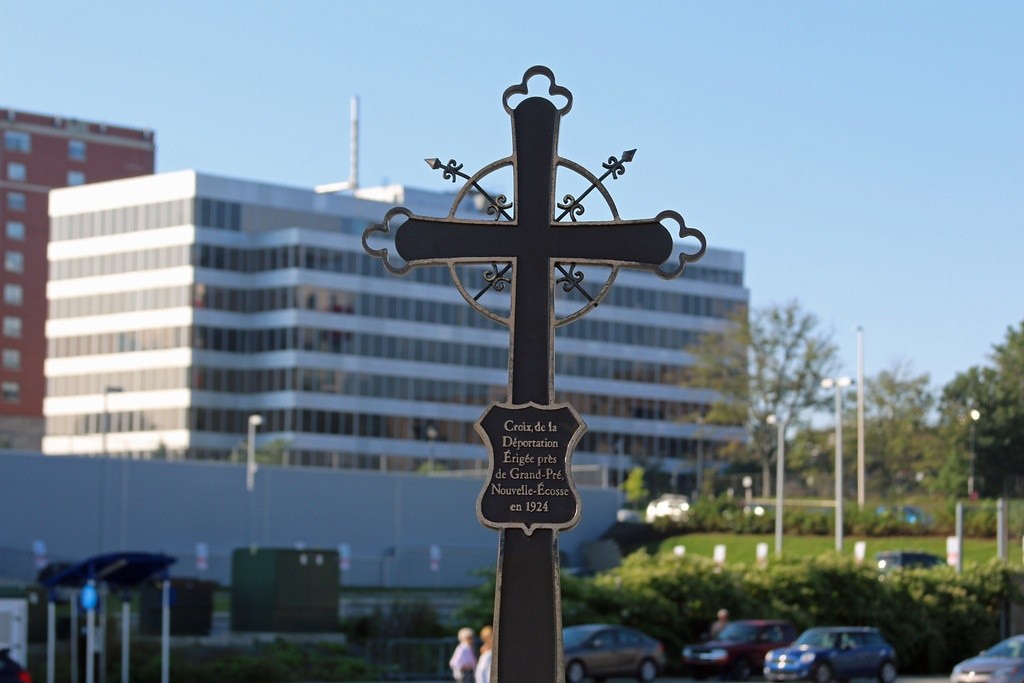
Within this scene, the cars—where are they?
[950,635,1024,683]
[562,624,668,683]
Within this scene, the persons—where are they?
[449,625,493,683]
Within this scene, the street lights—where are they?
[247,414,263,548]
[821,378,852,551]
[767,413,789,563]
[99,385,124,555]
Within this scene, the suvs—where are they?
[682,619,798,682]
[764,625,897,683]
